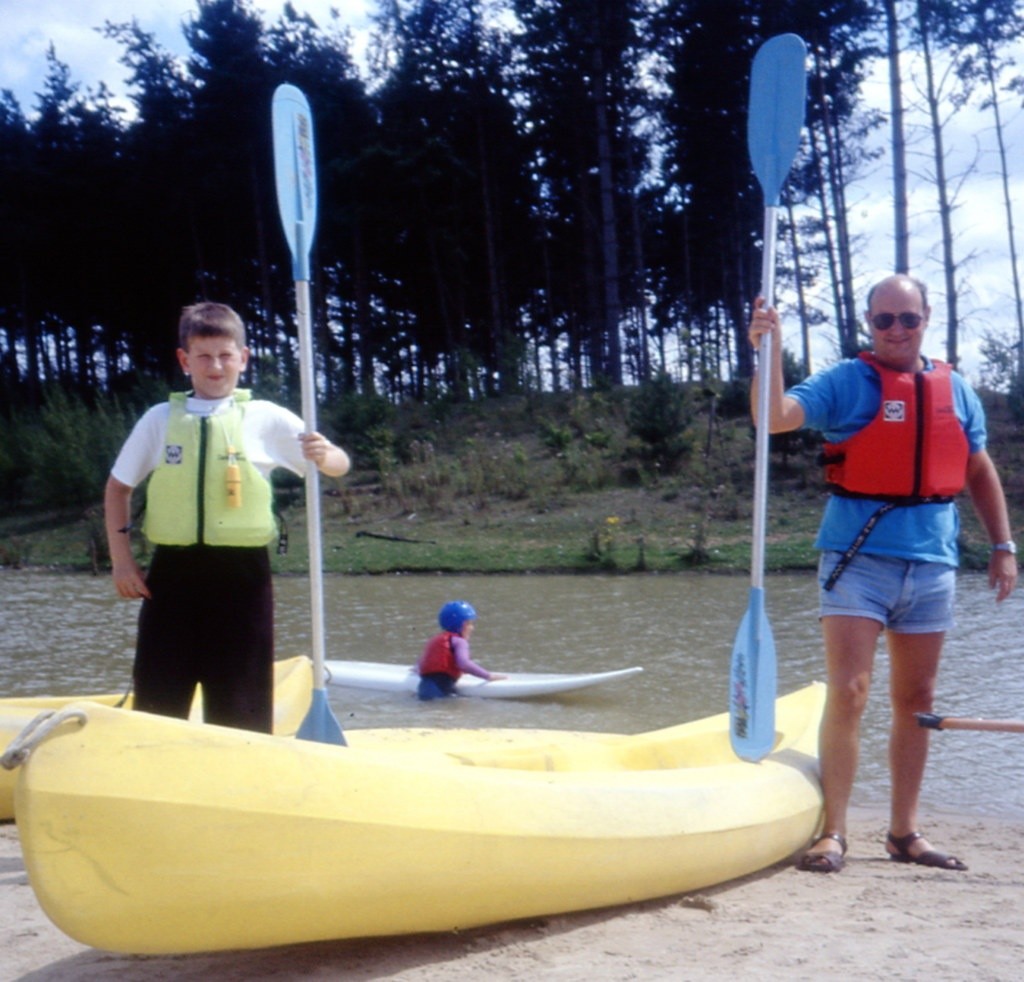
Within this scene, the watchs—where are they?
[991,541,1017,554]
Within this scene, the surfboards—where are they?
[320,653,645,698]
[0,653,316,823]
[0,680,833,957]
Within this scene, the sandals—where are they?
[885,830,969,871]
[796,832,847,872]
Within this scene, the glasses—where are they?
[869,311,925,330]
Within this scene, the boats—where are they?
[322,660,643,698]
[0,679,835,958]
[0,655,316,822]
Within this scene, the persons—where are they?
[105,304,352,736]
[415,599,508,701]
[748,273,1020,876]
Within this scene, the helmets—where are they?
[438,599,475,635]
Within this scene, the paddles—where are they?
[271,82,346,753]
[730,32,809,762]
